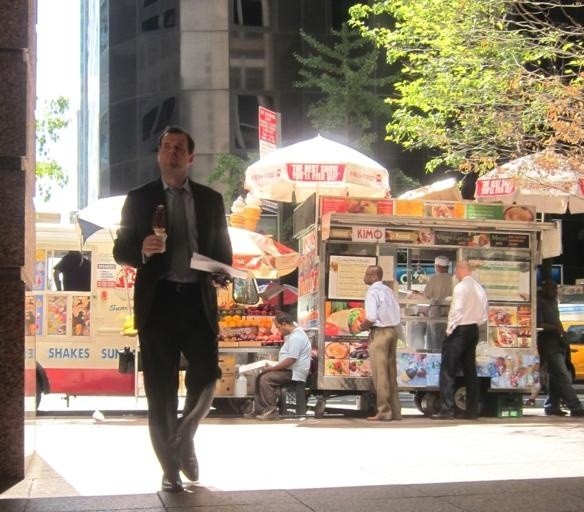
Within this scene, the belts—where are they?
[159,282,200,296]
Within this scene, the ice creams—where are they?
[152,205,167,252]
[230,196,244,229]
[244,192,263,231]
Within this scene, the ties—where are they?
[171,188,190,280]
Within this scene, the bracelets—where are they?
[359,324,364,330]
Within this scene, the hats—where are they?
[435,256,449,266]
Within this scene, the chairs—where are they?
[278,352,314,415]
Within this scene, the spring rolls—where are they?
[328,307,366,335]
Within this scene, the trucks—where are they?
[34,212,273,412]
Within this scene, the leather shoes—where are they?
[162,474,183,492]
[177,418,198,481]
[432,411,455,419]
[455,412,477,419]
[368,415,402,421]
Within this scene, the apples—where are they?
[217,304,276,317]
[218,331,284,346]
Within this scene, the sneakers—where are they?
[243,410,261,419]
[256,409,276,419]
[546,410,567,416]
[571,410,584,416]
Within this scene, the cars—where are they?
[556,303,584,385]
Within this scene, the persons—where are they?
[113,127,232,493]
[430,260,490,420]
[424,256,454,353]
[358,265,402,421]
[537,280,584,416]
[243,312,311,421]
[53,251,90,292]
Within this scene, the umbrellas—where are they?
[474,144,584,242]
[227,226,299,279]
[244,133,391,230]
